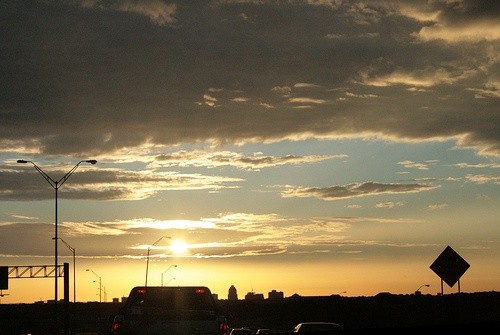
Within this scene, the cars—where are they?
[229,323,344,335]
[111,287,231,335]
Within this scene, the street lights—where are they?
[85,269,107,303]
[162,265,177,287]
[52,237,76,311]
[145,236,171,287]
[16,159,98,335]
